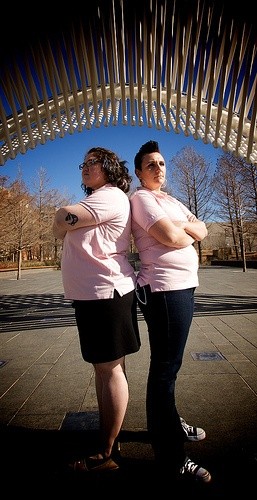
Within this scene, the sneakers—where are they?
[177,456,211,483]
[179,417,206,441]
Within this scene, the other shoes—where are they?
[73,451,112,466]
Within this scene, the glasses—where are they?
[79,159,103,170]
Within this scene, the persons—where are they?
[129,140,212,483]
[52,147,142,473]
